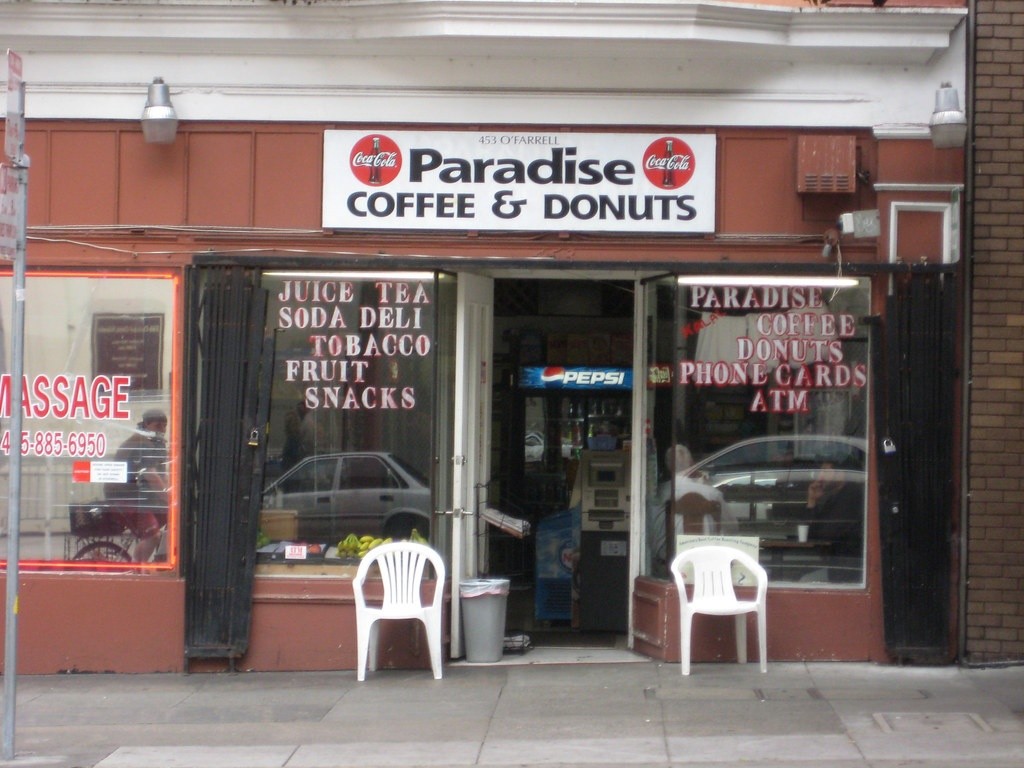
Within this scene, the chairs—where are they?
[671,546,768,675]
[352,542,446,682]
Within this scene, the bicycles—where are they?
[73,486,174,562]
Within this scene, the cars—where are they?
[674,433,868,552]
[262,451,431,538]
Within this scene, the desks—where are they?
[761,537,831,584]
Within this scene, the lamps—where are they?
[140,75,179,144]
[928,81,968,149]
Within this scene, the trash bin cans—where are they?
[459,579,509,664]
